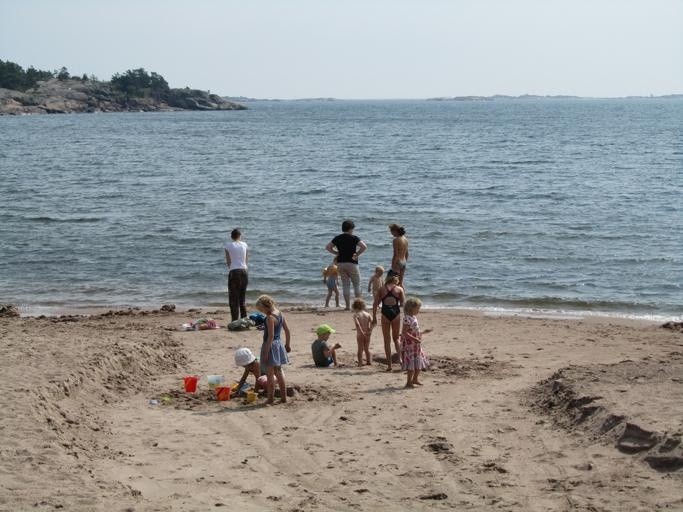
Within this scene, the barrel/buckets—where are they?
[184,376,198,392]
[208,375,224,389]
[247,390,258,402]
[216,385,230,400]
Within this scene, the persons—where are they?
[233,349,278,398]
[255,295,291,404]
[226,229,247,323]
[311,220,430,386]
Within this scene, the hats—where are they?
[316,324,336,336]
[233,347,256,366]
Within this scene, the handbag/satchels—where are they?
[227,312,267,331]
